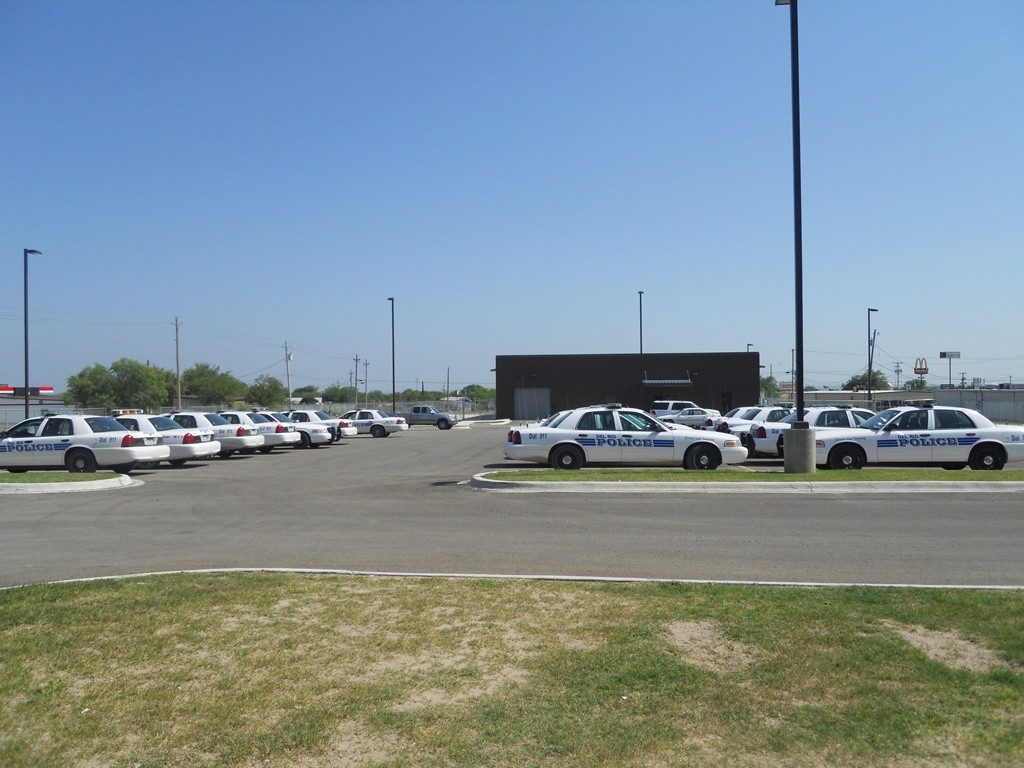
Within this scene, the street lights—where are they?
[867,307,879,400]
[747,344,760,353]
[775,0,813,428]
[22,247,43,420]
[638,291,645,410]
[387,297,395,413]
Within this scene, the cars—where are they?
[110,408,221,469]
[280,410,358,445]
[255,411,336,449]
[215,409,302,453]
[336,408,409,438]
[500,398,1024,472]
[0,411,171,474]
[156,410,265,459]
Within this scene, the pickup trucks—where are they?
[385,403,458,430]
